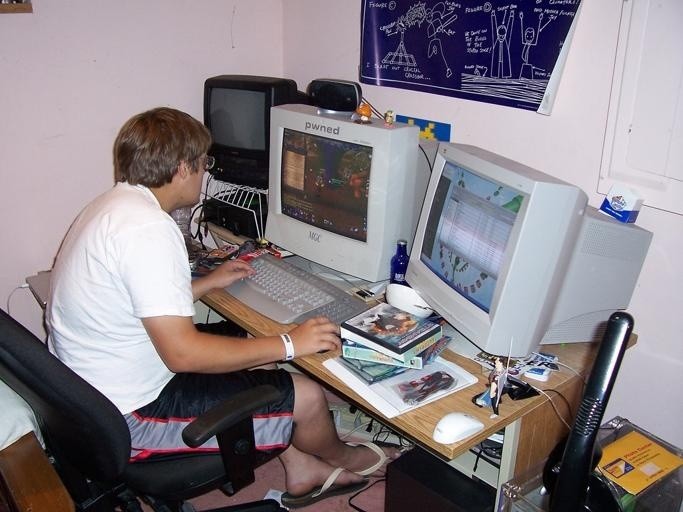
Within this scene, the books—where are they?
[339,356,404,380]
[336,303,445,361]
[339,335,453,369]
[476,350,559,383]
[335,360,407,385]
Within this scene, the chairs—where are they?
[0,306,290,512]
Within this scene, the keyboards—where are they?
[224,253,370,333]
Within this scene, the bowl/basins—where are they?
[384,284,435,319]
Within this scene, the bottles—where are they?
[172,205,191,235]
[390,240,410,288]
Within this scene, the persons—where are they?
[475,355,509,418]
[45,108,400,506]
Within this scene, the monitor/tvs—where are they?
[404,143,653,357]
[204,74,297,192]
[265,104,438,284]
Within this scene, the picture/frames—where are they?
[0,1,33,15]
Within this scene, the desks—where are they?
[190,215,637,512]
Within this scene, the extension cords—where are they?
[331,405,411,446]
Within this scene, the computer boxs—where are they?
[385,447,497,512]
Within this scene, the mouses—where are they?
[432,410,486,446]
[315,331,346,354]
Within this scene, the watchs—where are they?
[278,331,296,361]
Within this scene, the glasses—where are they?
[178,156,216,170]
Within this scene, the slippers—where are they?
[344,440,408,477]
[280,466,370,509]
